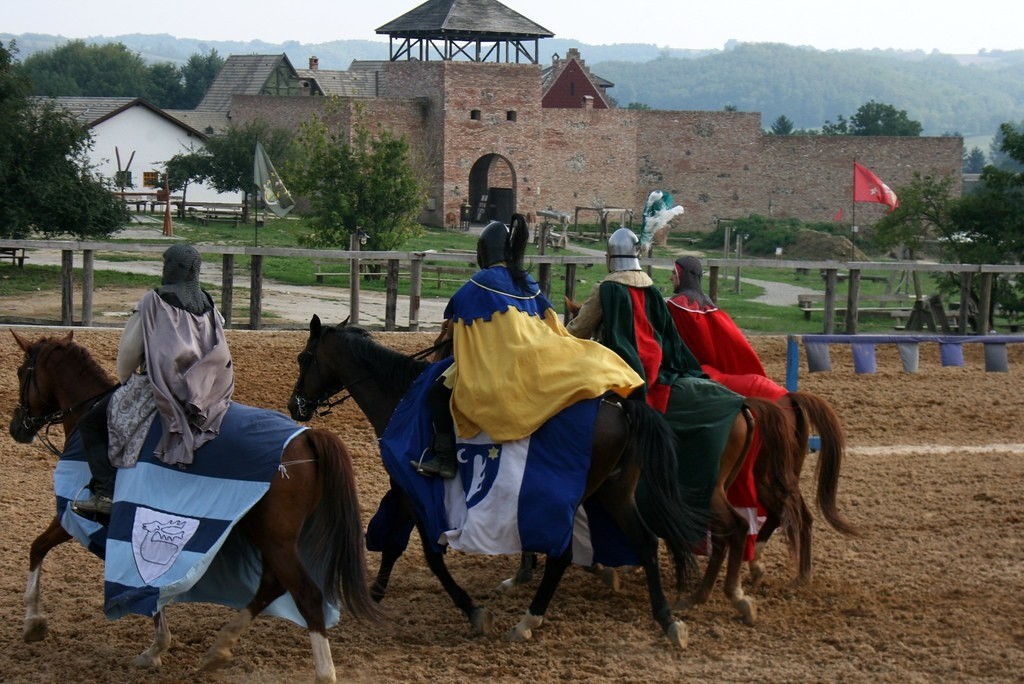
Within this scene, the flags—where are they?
[854,160,899,216]
[254,140,296,220]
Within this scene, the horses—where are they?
[285,298,866,650]
[9,329,390,684]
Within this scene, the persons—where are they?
[565,227,682,399]
[408,221,560,480]
[71,242,234,528]
[664,255,758,395]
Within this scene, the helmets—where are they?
[474,219,513,267]
[606,228,644,276]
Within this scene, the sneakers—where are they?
[67,493,113,518]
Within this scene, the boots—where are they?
[413,430,459,479]
